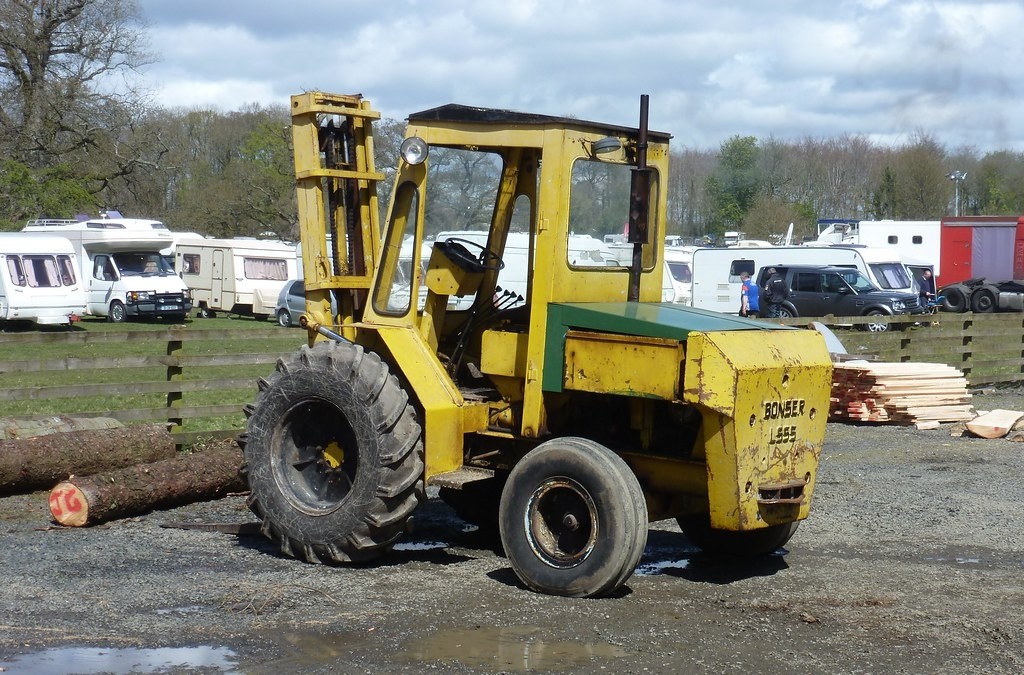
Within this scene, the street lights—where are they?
[945,171,968,217]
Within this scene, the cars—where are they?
[276,280,339,328]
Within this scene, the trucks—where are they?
[22,210,192,324]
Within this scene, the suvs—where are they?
[755,264,922,333]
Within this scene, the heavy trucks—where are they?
[938,216,1024,314]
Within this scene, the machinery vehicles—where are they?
[234,92,833,599]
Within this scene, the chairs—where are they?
[143,262,159,273]
[821,276,827,293]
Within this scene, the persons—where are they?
[763,268,791,318]
[917,270,932,327]
[739,271,759,318]
